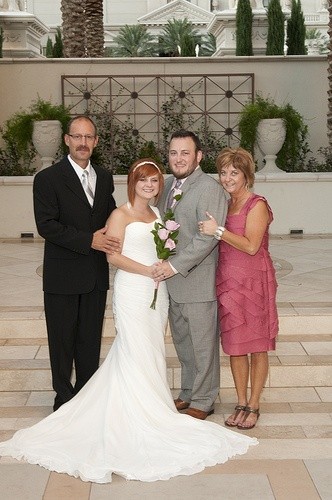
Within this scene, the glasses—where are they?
[67,133,95,141]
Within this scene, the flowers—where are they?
[170,187,184,208]
[149,208,181,310]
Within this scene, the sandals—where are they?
[225,405,247,427]
[238,407,260,430]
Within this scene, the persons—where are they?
[0,158,259,483]
[199,147,279,430]
[33,116,121,411]
[151,130,228,420]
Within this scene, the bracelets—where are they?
[214,226,225,240]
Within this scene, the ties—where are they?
[166,180,181,211]
[82,170,93,198]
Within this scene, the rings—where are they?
[200,228,202,231]
[209,216,212,218]
[200,221,202,224]
[162,273,165,278]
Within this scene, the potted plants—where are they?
[3,92,72,176]
[238,93,308,173]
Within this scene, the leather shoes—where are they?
[187,407,214,420]
[173,398,191,410]
